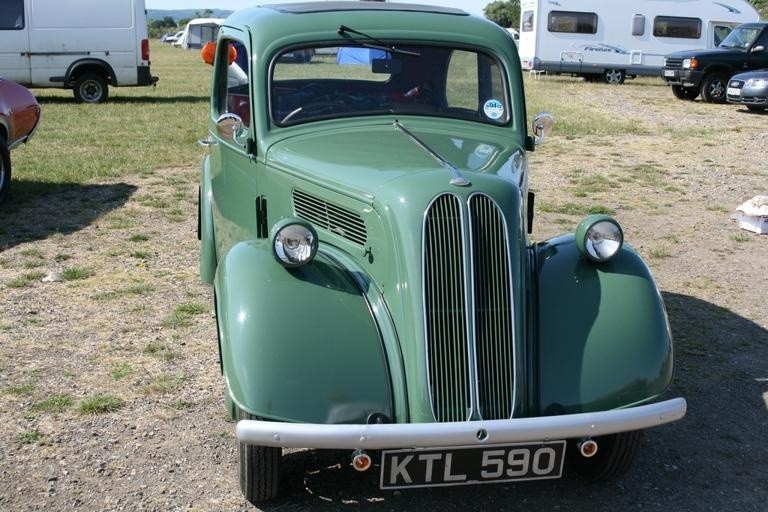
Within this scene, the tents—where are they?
[336,47,394,68]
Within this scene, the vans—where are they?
[517,1,767,87]
[0,0,160,103]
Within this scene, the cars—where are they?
[0,77,41,205]
[660,23,768,112]
[199,1,689,503]
[168,31,185,42]
